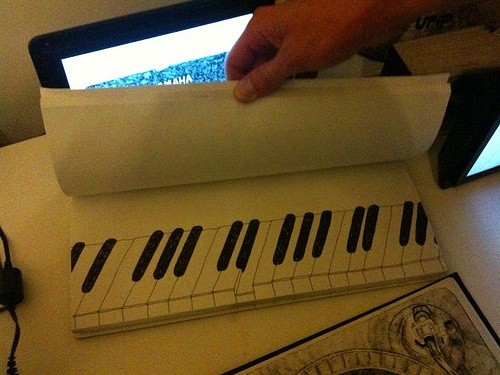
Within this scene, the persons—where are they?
[222,1,470,103]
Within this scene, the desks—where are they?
[2,18,500,375]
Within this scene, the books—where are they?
[34,69,451,343]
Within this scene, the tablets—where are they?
[28,1,279,88]
